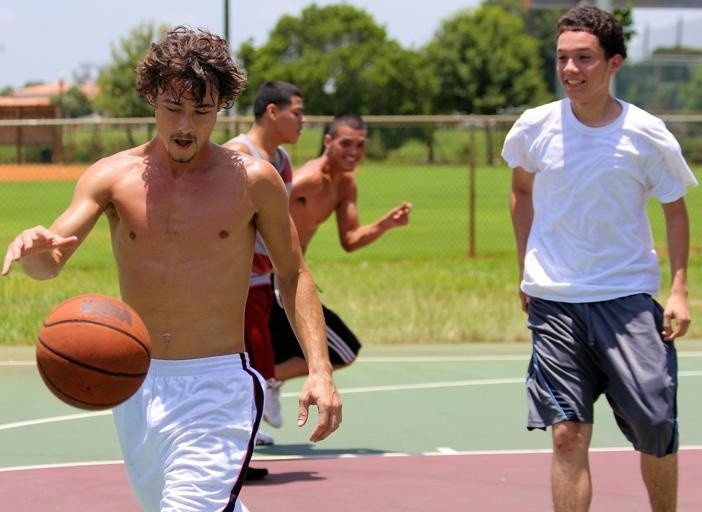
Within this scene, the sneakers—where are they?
[246,378,286,482]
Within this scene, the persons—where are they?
[219,79,306,480]
[254,115,411,445]
[500,5,698,512]
[0,25,342,512]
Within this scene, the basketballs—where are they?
[37,295,151,409]
